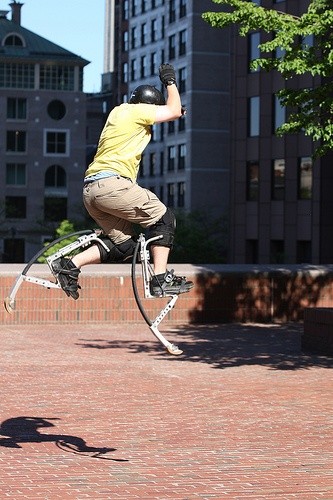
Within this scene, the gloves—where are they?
[159,64,177,88]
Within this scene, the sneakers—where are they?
[51,258,83,300]
[149,268,194,296]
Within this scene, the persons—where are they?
[53,63,193,300]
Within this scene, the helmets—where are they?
[130,85,167,106]
